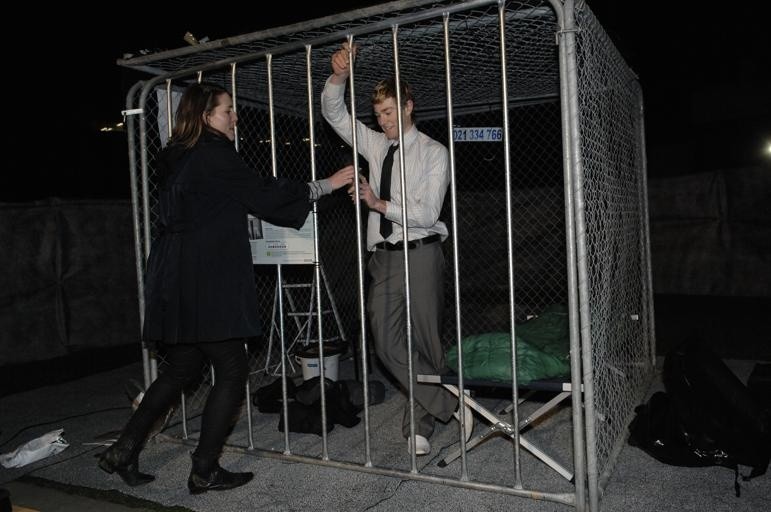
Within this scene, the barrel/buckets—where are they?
[295,342,343,382]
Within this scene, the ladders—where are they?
[263,254,347,376]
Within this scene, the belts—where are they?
[375,236,440,251]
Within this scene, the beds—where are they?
[417,323,642,488]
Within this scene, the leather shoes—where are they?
[408,434,430,456]
[455,388,474,443]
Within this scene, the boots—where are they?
[99,430,156,486]
[189,450,253,495]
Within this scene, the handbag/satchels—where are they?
[629,339,771,497]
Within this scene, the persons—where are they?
[319,43,474,456]
[0,486,40,512]
[97,77,362,496]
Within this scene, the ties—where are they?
[380,143,396,239]
